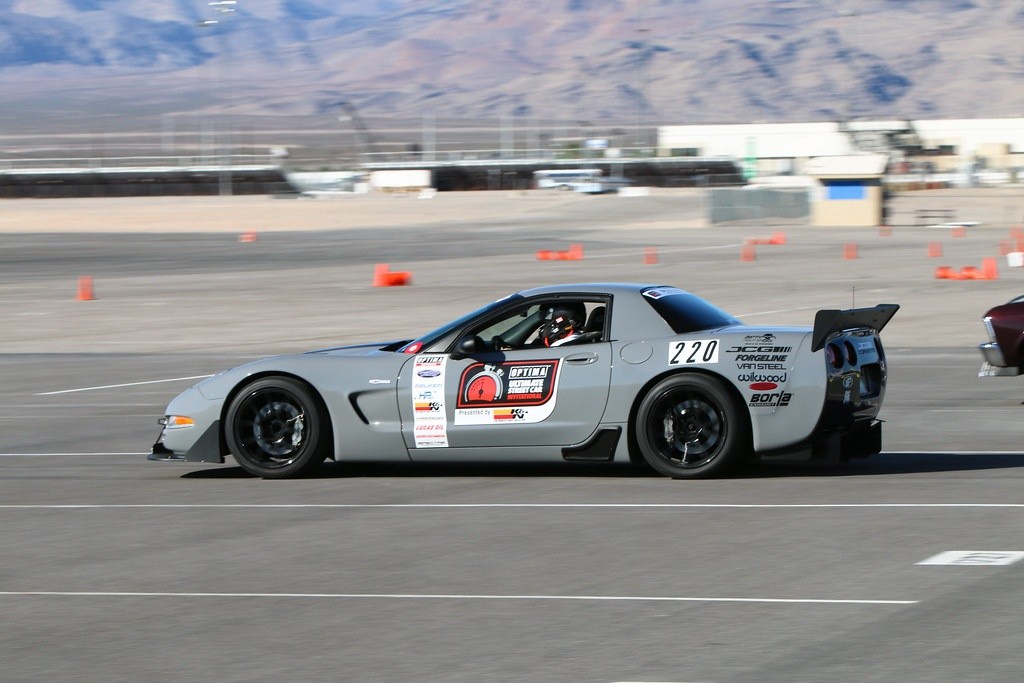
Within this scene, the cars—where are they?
[977,295,1024,378]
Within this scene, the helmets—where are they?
[537,299,586,343]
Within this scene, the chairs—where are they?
[586,306,606,341]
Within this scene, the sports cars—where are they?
[147,283,901,482]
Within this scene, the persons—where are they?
[500,300,586,350]
